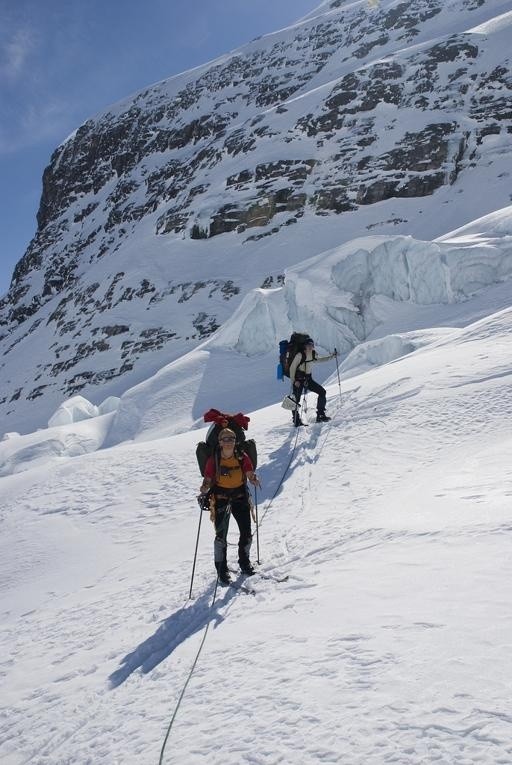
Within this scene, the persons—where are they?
[196,427,262,587]
[289,338,337,426]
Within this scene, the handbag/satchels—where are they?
[281,395,302,411]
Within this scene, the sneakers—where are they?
[317,412,331,422]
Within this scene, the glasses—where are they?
[218,437,235,442]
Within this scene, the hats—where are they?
[218,428,236,446]
[304,339,313,345]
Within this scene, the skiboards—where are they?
[225,569,289,595]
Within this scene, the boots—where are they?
[215,560,234,588]
[293,419,308,427]
[237,559,256,576]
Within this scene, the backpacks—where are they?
[283,332,317,378]
[196,414,257,482]
